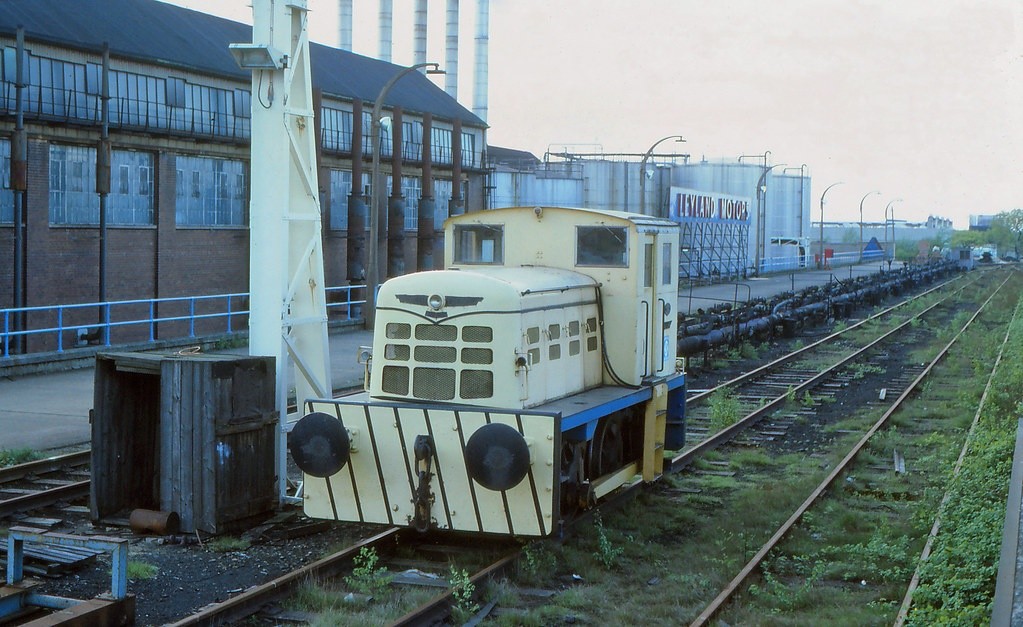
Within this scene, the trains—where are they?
[289,205,684,540]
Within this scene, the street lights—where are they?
[859,190,882,264]
[756,163,789,277]
[819,180,847,268]
[884,198,903,255]
[364,62,447,331]
[638,135,687,215]
